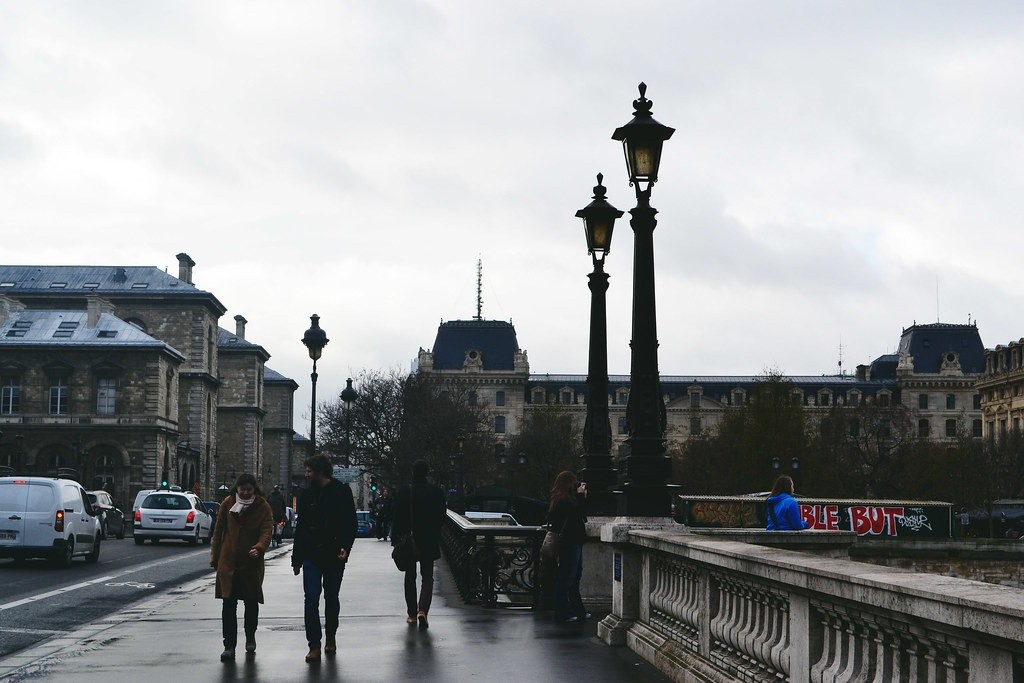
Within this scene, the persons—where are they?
[209,473,274,661]
[389,460,447,628]
[291,453,360,661]
[548,471,592,622]
[766,475,808,530]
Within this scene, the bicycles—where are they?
[272,515,287,547]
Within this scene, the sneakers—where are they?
[324,632,336,653]
[306,648,321,662]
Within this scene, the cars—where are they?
[85,490,126,539]
[356,521,372,538]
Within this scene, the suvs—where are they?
[132,486,221,546]
[0,466,102,569]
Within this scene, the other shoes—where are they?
[583,613,592,619]
[565,616,578,622]
[377,536,381,539]
[245,630,256,651]
[418,609,428,629]
[407,616,417,623]
[384,537,387,540]
[220,646,235,661]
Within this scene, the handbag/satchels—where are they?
[540,531,562,559]
[392,542,414,571]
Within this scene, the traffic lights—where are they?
[371,477,377,491]
[161,471,169,490]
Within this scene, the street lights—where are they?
[457,432,466,515]
[301,313,330,457]
[499,449,526,513]
[576,173,625,516]
[339,378,359,468]
[611,82,676,518]
